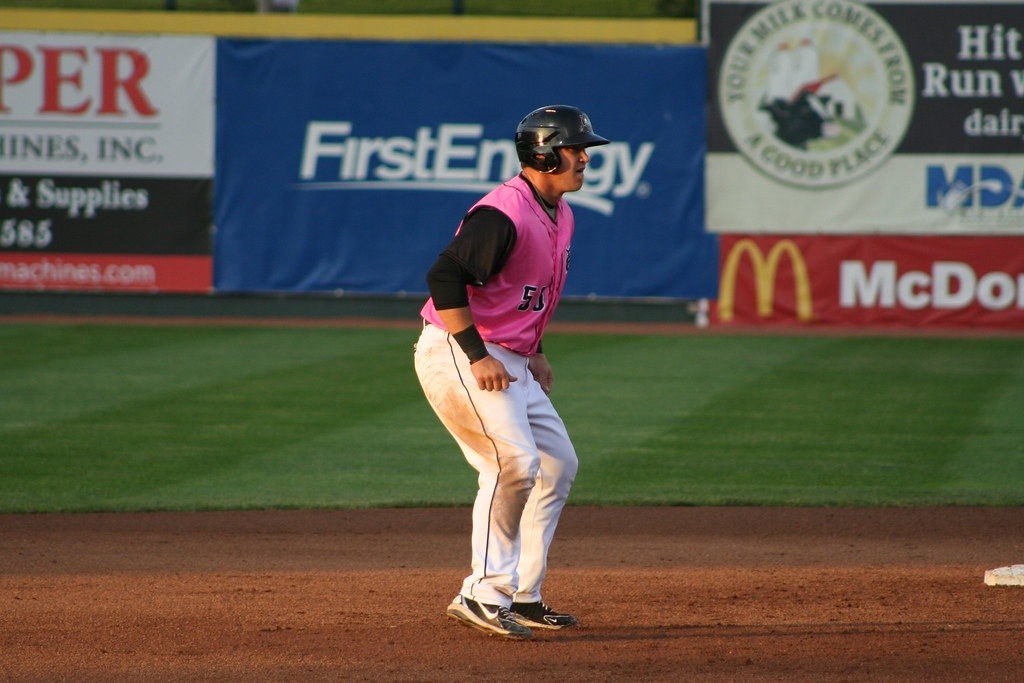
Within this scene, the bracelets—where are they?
[452,323,490,365]
[536,340,543,354]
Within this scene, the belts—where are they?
[424,318,431,326]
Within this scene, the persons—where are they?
[412,104,609,640]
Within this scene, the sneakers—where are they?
[509,600,576,629]
[447,594,532,641]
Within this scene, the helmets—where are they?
[514,104,611,174]
